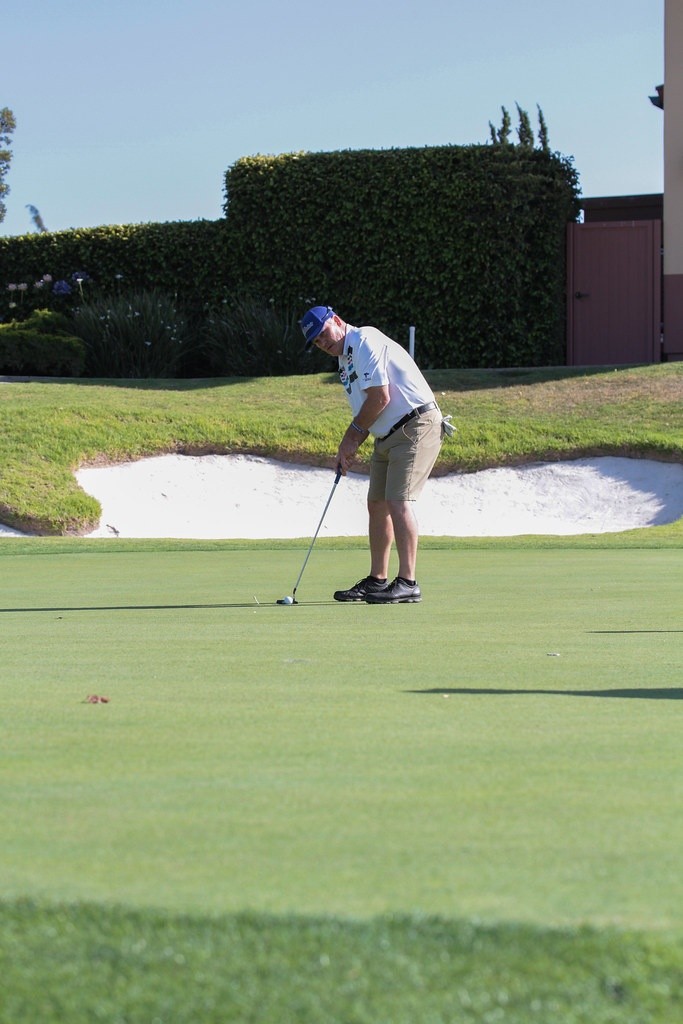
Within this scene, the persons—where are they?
[298,306,455,604]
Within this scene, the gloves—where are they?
[443,414,458,437]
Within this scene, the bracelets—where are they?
[351,422,366,434]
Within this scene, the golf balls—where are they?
[282,596,295,606]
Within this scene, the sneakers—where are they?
[333,577,390,602]
[365,577,424,604]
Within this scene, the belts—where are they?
[381,402,436,441]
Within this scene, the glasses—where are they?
[321,306,333,321]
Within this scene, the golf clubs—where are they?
[277,429,371,606]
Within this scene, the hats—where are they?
[301,305,336,352]
